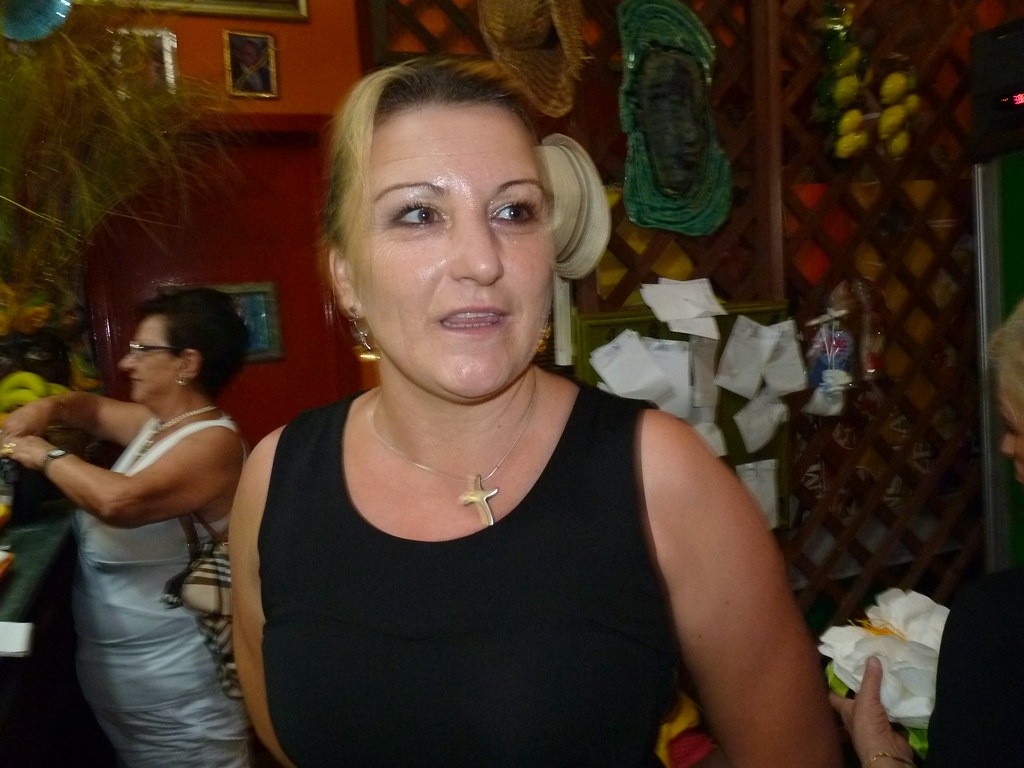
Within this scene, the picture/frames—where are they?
[111,28,177,105]
[71,0,310,25]
[205,282,284,365]
[222,27,280,101]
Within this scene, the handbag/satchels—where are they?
[164,424,257,701]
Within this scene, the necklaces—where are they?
[370,368,540,527]
[132,405,218,462]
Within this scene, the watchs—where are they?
[38,445,73,474]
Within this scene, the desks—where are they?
[0,452,118,662]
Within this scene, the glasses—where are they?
[128,340,184,361]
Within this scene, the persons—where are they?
[825,294,1023,767]
[228,53,854,767]
[0,284,257,767]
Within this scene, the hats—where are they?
[616,0,735,235]
[535,132,611,279]
[477,0,589,117]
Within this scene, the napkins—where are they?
[0,621,34,658]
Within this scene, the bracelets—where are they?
[863,752,914,767]
